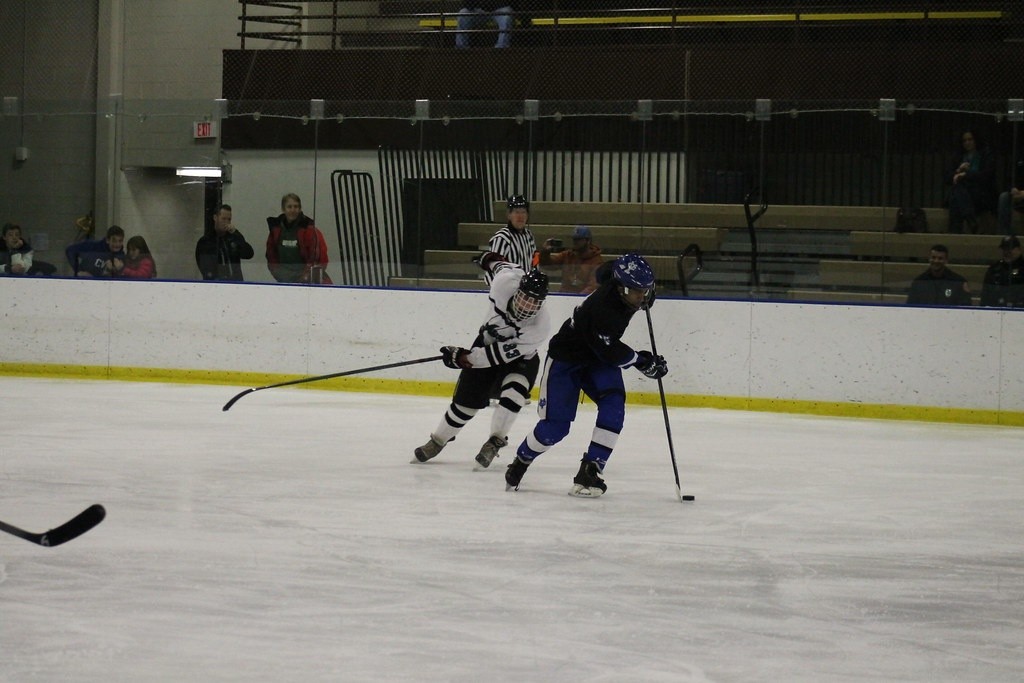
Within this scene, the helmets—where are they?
[613,252,654,309]
[513,270,549,321]
[507,195,529,209]
[573,226,593,240]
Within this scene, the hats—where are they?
[998,235,1020,248]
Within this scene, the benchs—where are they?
[419,10,1001,26]
[387,200,1024,301]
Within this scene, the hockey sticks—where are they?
[222,355,444,412]
[645,304,689,504]
[0,504,107,547]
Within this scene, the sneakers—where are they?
[408,433,456,465]
[471,432,510,473]
[504,452,533,493]
[568,451,607,496]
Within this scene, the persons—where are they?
[65,226,157,278]
[947,129,1024,235]
[455,0,532,48]
[906,244,974,306]
[266,193,333,285]
[195,205,254,282]
[484,195,541,288]
[540,226,605,294]
[505,253,668,494]
[414,250,550,467]
[980,235,1024,308]
[0,224,33,275]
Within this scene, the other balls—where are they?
[682,495,695,501]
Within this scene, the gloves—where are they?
[477,250,504,270]
[439,346,470,370]
[640,350,668,380]
[644,286,655,310]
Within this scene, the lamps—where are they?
[175,165,232,186]
